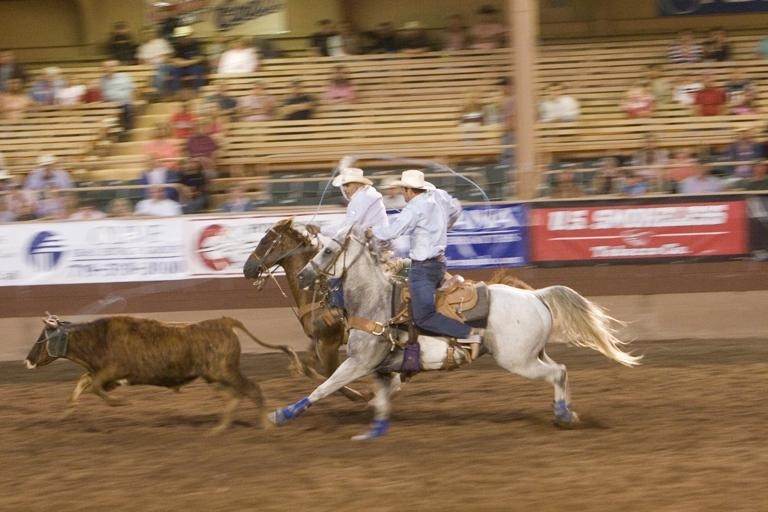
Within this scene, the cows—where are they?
[22,309,304,437]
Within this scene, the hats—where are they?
[36,154,58,168]
[390,170,437,192]
[397,20,427,33]
[491,74,512,88]
[170,25,194,39]
[0,169,16,181]
[331,167,374,187]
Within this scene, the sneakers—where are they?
[468,326,485,360]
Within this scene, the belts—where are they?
[433,254,447,263]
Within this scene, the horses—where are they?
[262,224,645,441]
[242,217,536,403]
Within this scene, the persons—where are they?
[463,27,768,260]
[0,1,354,223]
[303,165,394,248]
[306,5,510,58]
[364,167,484,363]
